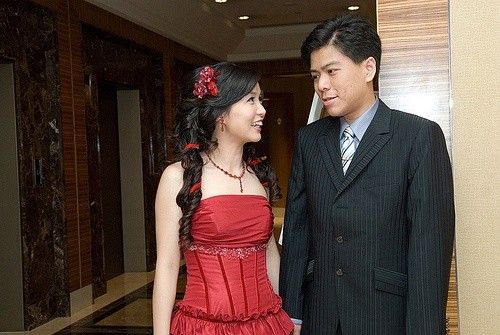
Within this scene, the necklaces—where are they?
[204,150,245,193]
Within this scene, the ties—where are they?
[340,126,356,177]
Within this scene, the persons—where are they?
[279,13,455,335]
[153,61,295,335]
